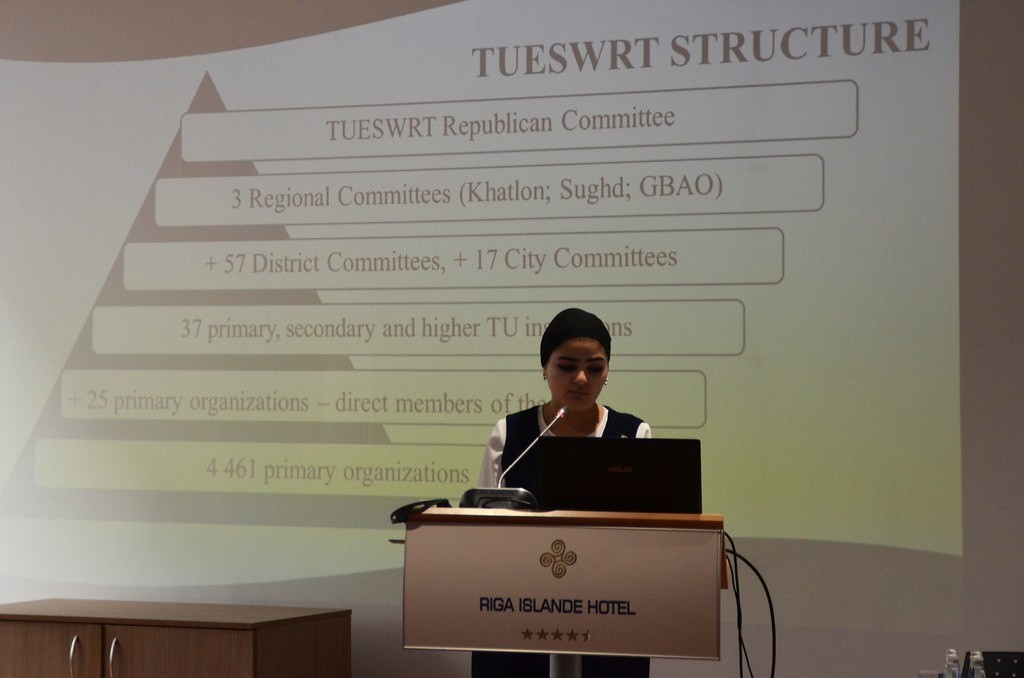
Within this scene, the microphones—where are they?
[459,406,571,512]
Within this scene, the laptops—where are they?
[539,437,702,515]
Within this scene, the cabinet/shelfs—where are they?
[0,598,352,678]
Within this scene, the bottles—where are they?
[967,650,986,678]
[943,648,962,678]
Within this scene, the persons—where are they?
[470,308,651,678]
